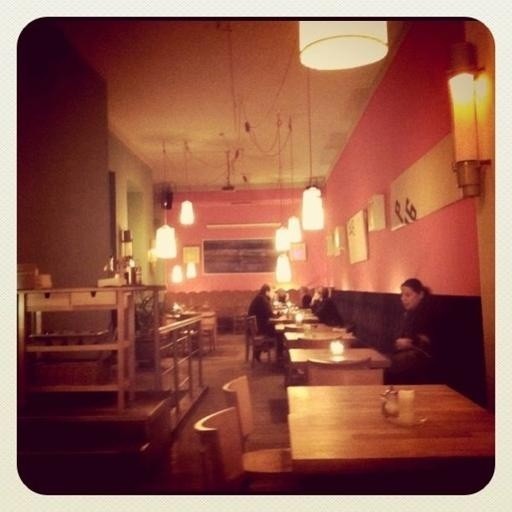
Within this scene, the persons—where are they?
[246,283,284,363]
[274,287,311,309]
[308,286,344,328]
[379,278,445,384]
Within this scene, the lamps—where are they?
[177,140,197,226]
[274,120,293,251]
[153,139,179,260]
[120,229,135,268]
[445,41,487,197]
[299,80,326,232]
[298,20,390,72]
[284,115,303,242]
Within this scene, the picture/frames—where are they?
[200,236,282,274]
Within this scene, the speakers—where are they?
[161,192,173,209]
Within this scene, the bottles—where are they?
[120,256,143,286]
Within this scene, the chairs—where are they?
[194,406,292,495]
[163,307,218,355]
[223,376,289,452]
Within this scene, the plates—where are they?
[387,411,426,427]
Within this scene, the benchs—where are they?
[324,289,489,406]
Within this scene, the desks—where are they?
[17,285,147,410]
[286,384,494,495]
